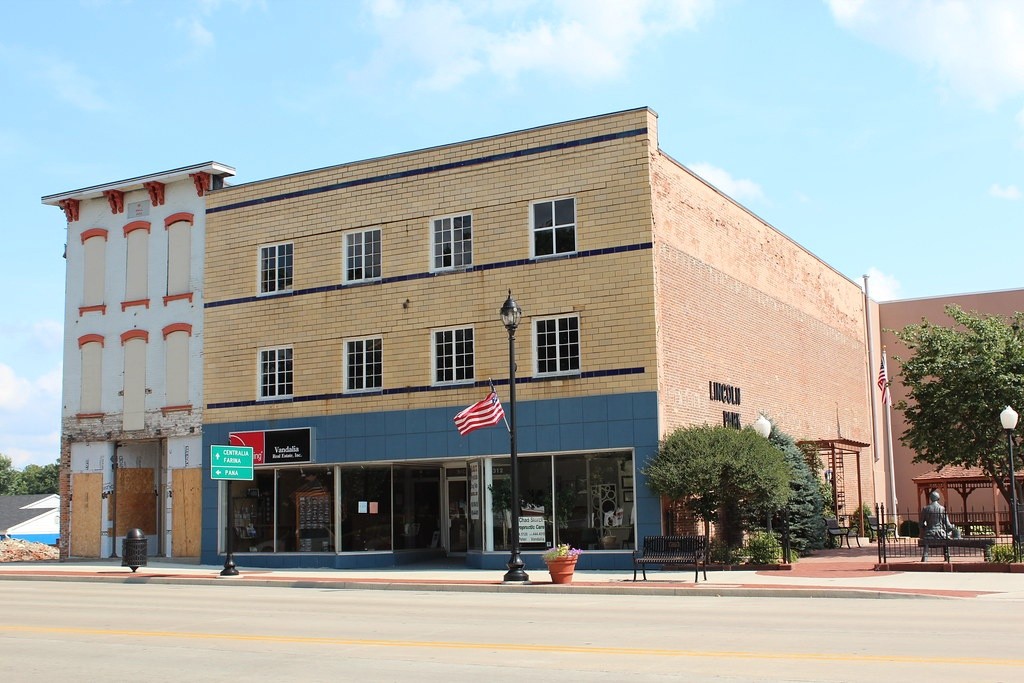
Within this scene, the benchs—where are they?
[633,536,708,583]
[918,538,995,562]
[867,515,898,543]
[823,517,860,549]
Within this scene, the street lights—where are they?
[500,289,529,581]
[1000,405,1020,560]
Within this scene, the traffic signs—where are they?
[210,444,254,480]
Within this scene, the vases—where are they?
[545,555,578,583]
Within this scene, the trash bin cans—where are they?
[121,528,148,573]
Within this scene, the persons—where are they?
[920,492,955,562]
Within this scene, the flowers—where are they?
[542,543,583,561]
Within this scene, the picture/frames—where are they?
[622,476,633,489]
[623,490,634,502]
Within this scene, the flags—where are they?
[877,359,891,407]
[454,391,505,435]
